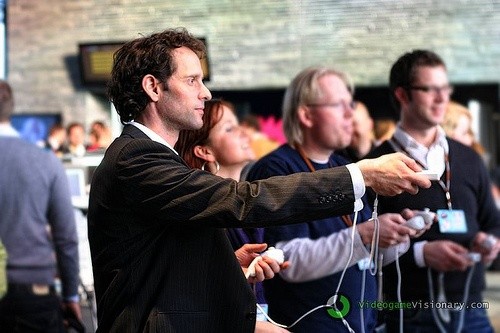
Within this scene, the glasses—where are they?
[410,85,453,94]
[306,99,355,109]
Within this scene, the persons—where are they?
[440,100,489,154]
[44,125,63,152]
[0,75,83,333]
[86,29,431,333]
[247,67,441,333]
[175,81,289,333]
[354,47,500,333]
[66,123,87,156]
[90,122,107,146]
[351,99,382,159]
